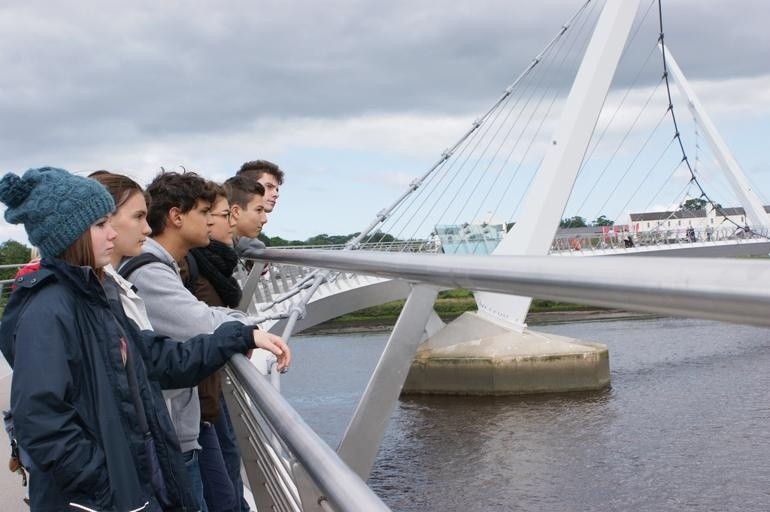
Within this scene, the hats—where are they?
[1,166,116,258]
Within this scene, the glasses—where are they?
[209,212,233,222]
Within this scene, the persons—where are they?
[1,165,292,512]
[175,180,244,512]
[223,177,268,237]
[86,169,172,417]
[118,166,252,512]
[233,160,284,254]
